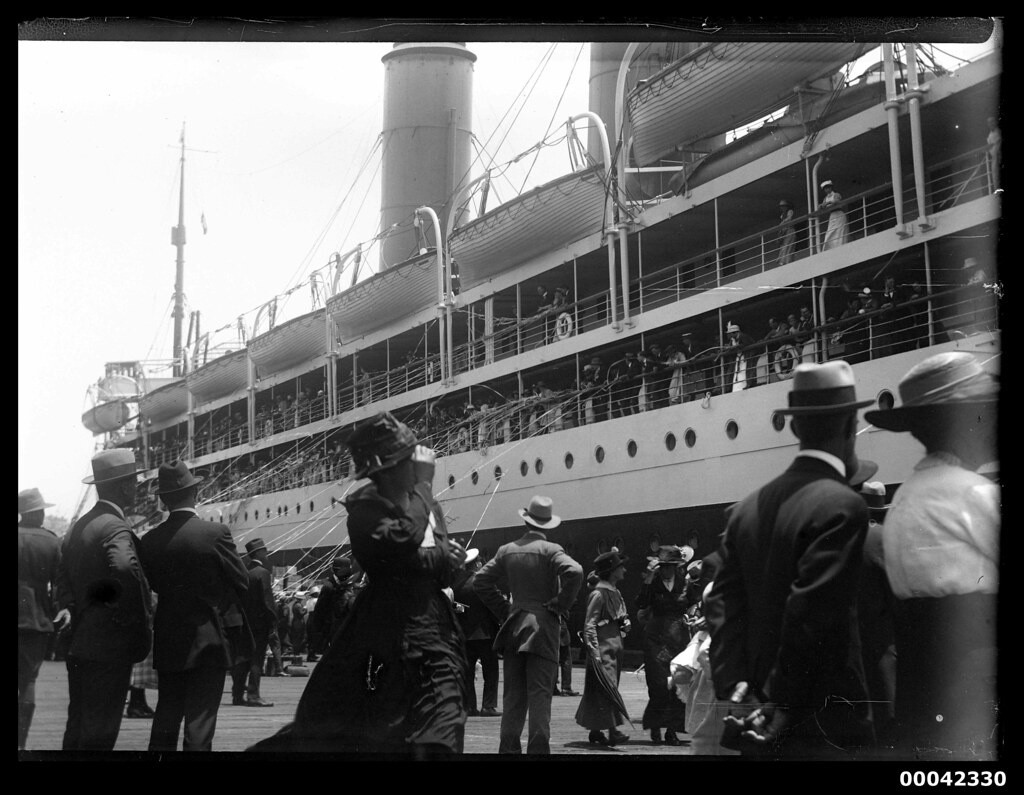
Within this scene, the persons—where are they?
[451,548,508,717]
[863,350,999,759]
[818,180,849,252]
[704,361,875,756]
[845,455,883,562]
[553,611,579,697]
[135,257,994,505]
[986,116,1000,155]
[637,544,740,755]
[230,538,273,707]
[142,459,256,751]
[473,496,584,754]
[577,548,635,748]
[53,446,157,751]
[19,488,60,748]
[777,199,795,266]
[266,558,355,677]
[127,591,157,718]
[244,409,469,755]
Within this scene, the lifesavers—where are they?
[556,312,573,340]
[457,427,469,452]
[774,344,800,381]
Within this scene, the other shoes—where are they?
[276,671,290,676]
[231,694,273,706]
[463,688,681,746]
[127,703,154,718]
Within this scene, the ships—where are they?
[65,41,997,672]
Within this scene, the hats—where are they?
[18,488,56,515]
[718,502,740,535]
[978,462,999,484]
[582,342,677,373]
[685,560,703,582]
[647,544,694,571]
[148,461,204,493]
[308,586,320,595]
[594,551,623,574]
[858,481,893,510]
[843,453,879,485]
[586,570,600,588]
[518,495,561,529]
[726,325,740,334]
[463,548,482,567]
[772,359,875,415]
[81,447,147,483]
[246,537,266,554]
[864,353,1002,432]
[347,412,417,480]
[651,546,686,569]
[328,557,354,572]
[294,592,306,599]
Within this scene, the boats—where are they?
[183,346,258,407]
[136,378,194,426]
[246,306,340,381]
[624,41,885,169]
[445,153,616,294]
[81,396,131,437]
[323,247,443,347]
